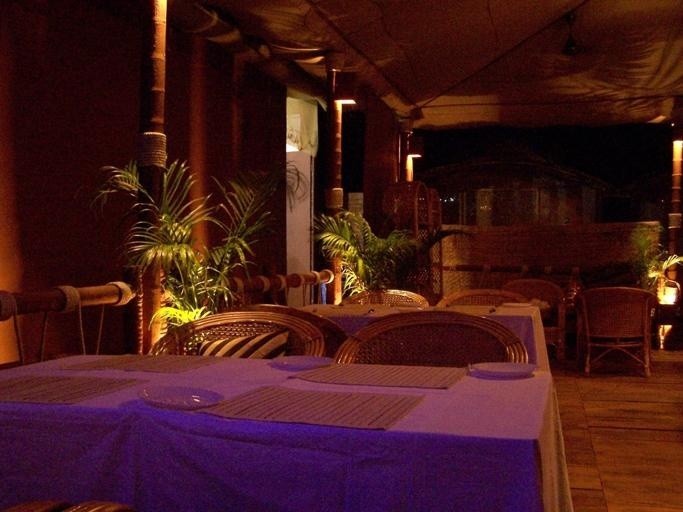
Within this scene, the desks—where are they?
[0,354,577,511]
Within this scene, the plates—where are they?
[468,363,535,378]
[137,385,225,410]
[272,356,332,369]
[503,303,532,307]
